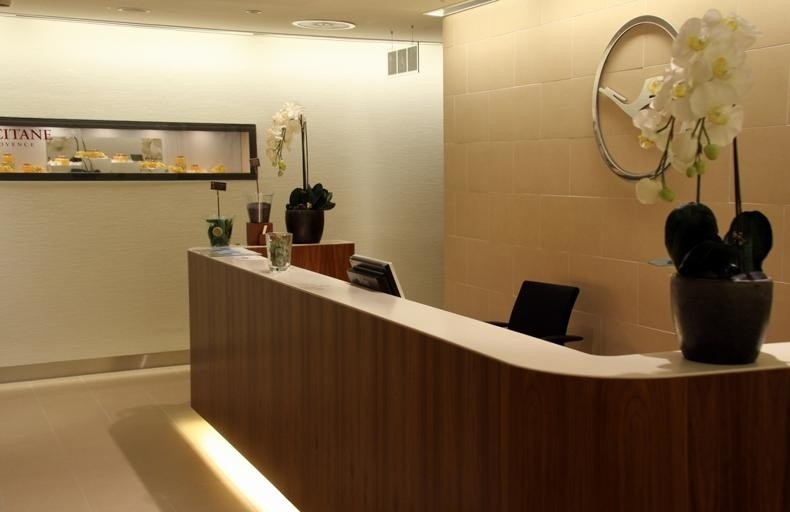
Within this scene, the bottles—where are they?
[175,156,186,173]
[190,164,199,172]
[0,154,15,172]
[22,163,32,172]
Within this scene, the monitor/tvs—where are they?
[345,253,404,297]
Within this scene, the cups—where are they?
[264,231,294,271]
[245,193,272,223]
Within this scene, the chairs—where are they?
[484,280,585,346]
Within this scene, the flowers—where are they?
[264,98,337,209]
[632,7,774,278]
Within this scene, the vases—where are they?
[245,191,276,222]
[668,278,776,366]
[206,218,235,249]
[283,209,325,245]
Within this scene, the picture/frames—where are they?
[0,116,258,181]
